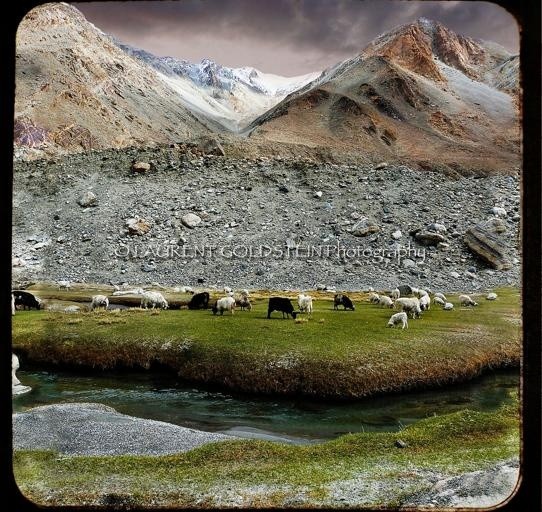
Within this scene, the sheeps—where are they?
[57,279,73,292]
[459,295,479,307]
[140,291,170,310]
[212,286,252,315]
[297,295,313,313]
[387,311,409,332]
[88,295,110,313]
[369,284,448,319]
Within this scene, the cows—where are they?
[11,291,45,311]
[267,297,297,319]
[333,294,355,311]
[187,291,210,310]
